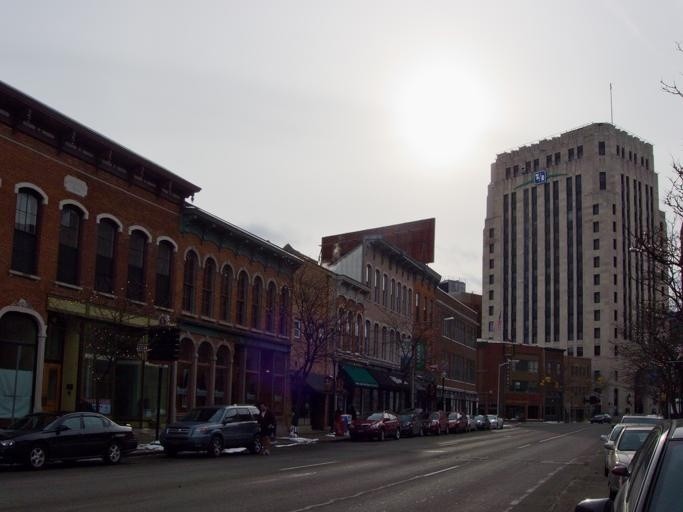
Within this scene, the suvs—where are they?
[158,403,265,456]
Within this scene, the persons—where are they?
[258,402,276,455]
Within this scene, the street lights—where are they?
[497,359,519,417]
[439,370,448,410]
[410,316,454,410]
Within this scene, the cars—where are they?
[0,410,137,473]
[348,406,504,442]
[575,412,683,511]
